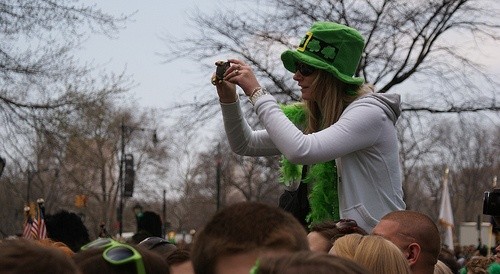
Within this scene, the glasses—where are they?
[336,218,358,235]
[81,237,145,274]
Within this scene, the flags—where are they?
[23,211,34,239]
[32,203,47,239]
[439,180,454,251]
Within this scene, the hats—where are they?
[281,22,365,86]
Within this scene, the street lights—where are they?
[26,168,61,206]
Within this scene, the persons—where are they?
[211,22,407,235]
[0,202,500,274]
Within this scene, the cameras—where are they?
[216,62,231,80]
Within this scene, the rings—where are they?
[237,64,241,70]
[235,69,238,75]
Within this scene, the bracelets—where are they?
[249,87,268,106]
